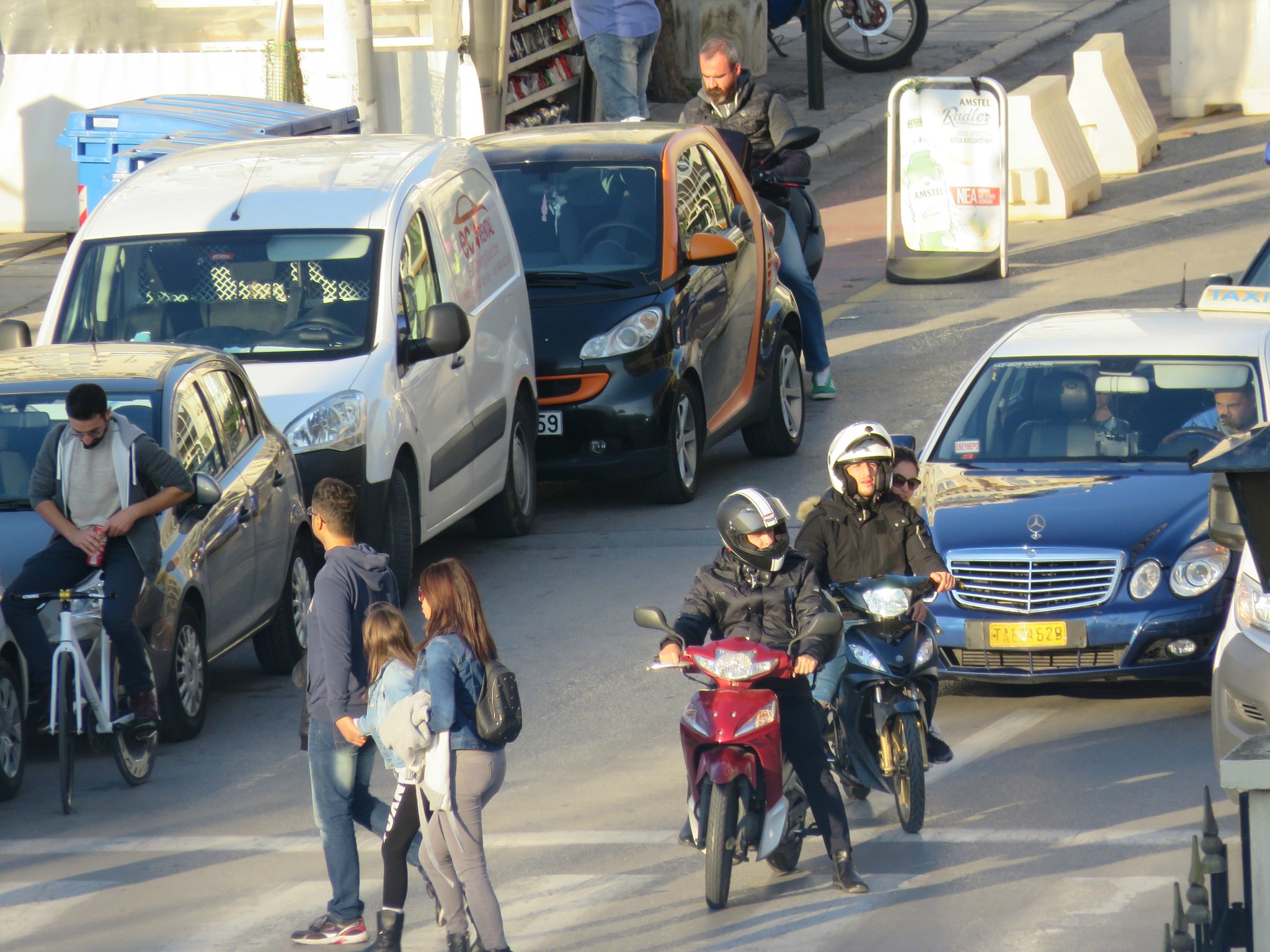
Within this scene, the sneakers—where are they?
[291,914,369,943]
[424,878,448,927]
[811,367,837,400]
[922,730,954,764]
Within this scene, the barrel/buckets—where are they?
[686,793,791,861]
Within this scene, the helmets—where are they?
[716,487,789,571]
[827,421,894,502]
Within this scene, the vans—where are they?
[0,135,540,604]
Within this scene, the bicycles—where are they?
[10,570,160,815]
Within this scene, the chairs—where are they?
[209,247,286,335]
[121,254,187,338]
[1008,372,1113,456]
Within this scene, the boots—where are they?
[361,910,404,951]
[465,906,488,952]
[678,816,695,845]
[447,931,470,952]
[831,851,869,893]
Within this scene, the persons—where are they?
[570,0,662,122]
[657,489,867,892]
[1081,365,1139,458]
[0,384,195,740]
[679,40,837,399]
[1182,382,1258,445]
[793,422,957,765]
[289,477,512,952]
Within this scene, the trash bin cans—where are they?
[54,94,361,228]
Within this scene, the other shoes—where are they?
[130,692,159,740]
[34,696,87,730]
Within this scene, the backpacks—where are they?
[442,628,524,743]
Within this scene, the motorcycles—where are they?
[767,0,930,73]
[633,605,844,909]
[811,569,965,834]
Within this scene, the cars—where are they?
[465,120,808,506]
[1,341,316,745]
[0,611,30,801]
[1203,236,1270,285]
[888,285,1270,697]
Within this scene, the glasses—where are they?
[69,413,106,437]
[418,586,425,601]
[306,507,326,525]
[891,473,922,491]
